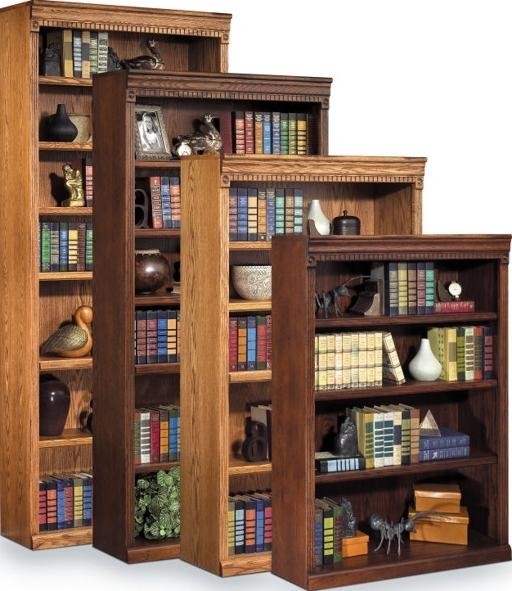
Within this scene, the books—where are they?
[229,489,274,555]
[82,151,95,207]
[38,218,96,275]
[36,471,93,534]
[232,109,314,157]
[314,262,493,473]
[134,174,181,467]
[228,313,274,374]
[314,506,342,568]
[228,184,304,243]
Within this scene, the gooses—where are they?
[120,33,166,70]
[176,112,224,152]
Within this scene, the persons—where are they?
[61,164,85,202]
[62,28,109,80]
[141,113,163,152]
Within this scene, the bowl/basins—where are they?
[231,265,272,301]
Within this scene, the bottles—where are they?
[39,374,72,437]
[406,335,443,383]
[135,246,171,293]
[306,198,331,235]
[48,102,79,142]
